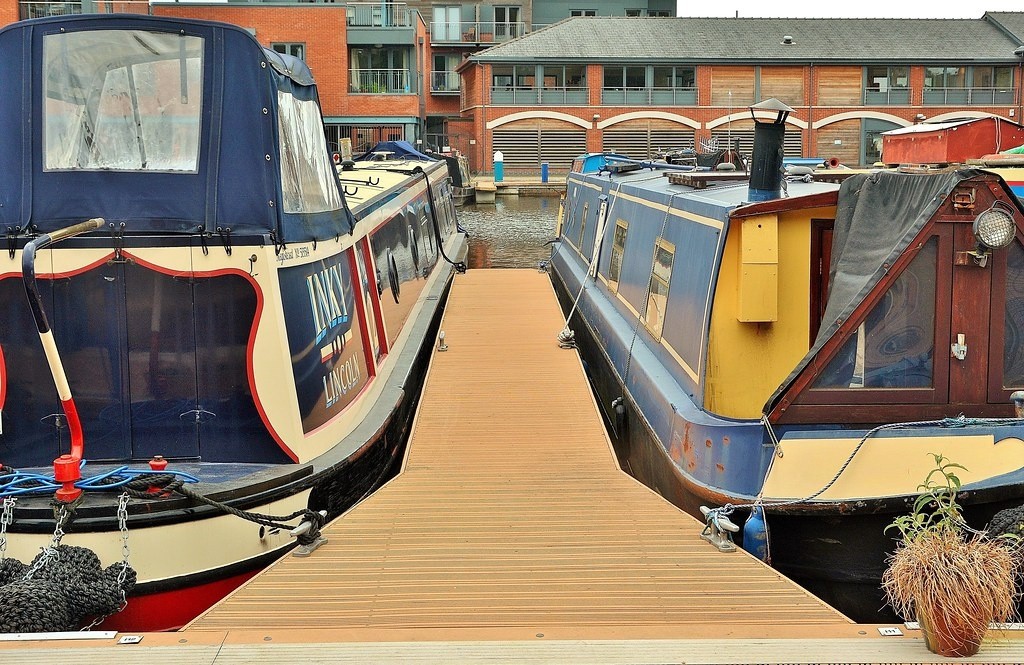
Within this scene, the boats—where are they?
[1,11,469,630]
[547,97,1022,588]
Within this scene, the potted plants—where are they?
[878,453,1024,657]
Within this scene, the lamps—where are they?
[972,200,1016,256]
